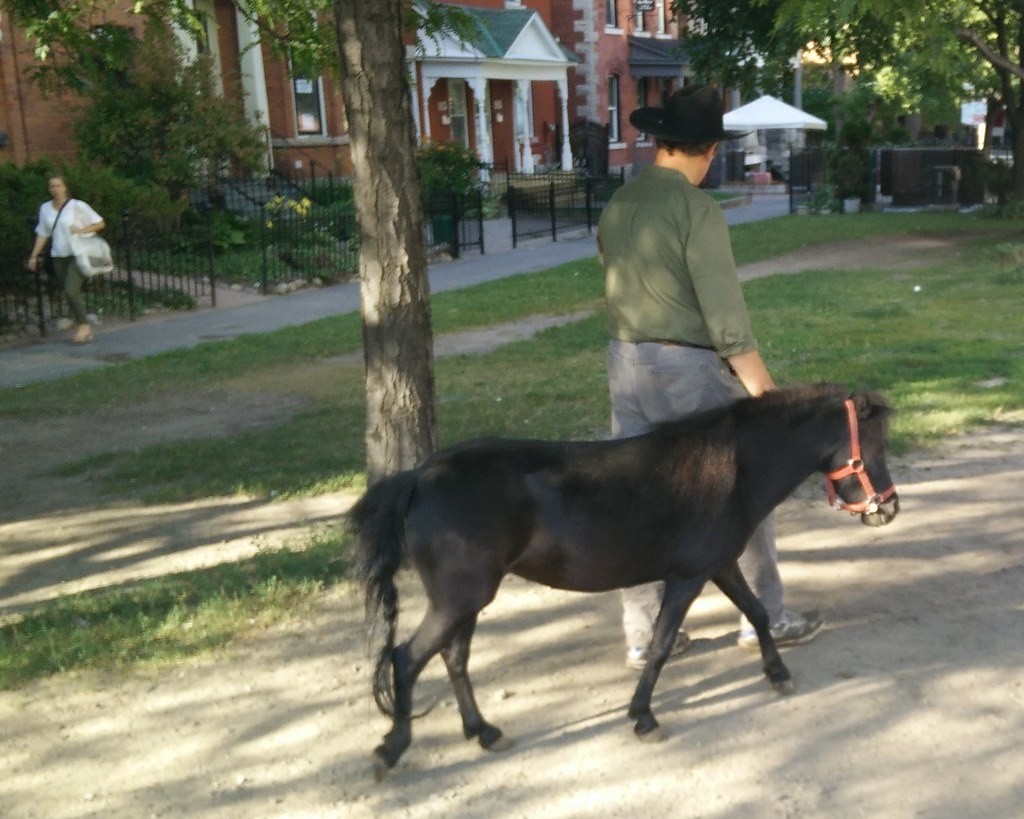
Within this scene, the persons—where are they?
[593,84,908,669]
[25,173,113,344]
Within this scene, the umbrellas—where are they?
[723,94,828,131]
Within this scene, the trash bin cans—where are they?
[931,165,961,204]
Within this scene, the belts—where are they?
[653,339,718,351]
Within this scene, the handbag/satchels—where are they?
[70,199,114,277]
[43,237,53,277]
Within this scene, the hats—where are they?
[629,83,755,144]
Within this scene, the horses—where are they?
[346,379,900,781]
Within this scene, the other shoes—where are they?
[74,328,95,343]
[58,317,74,331]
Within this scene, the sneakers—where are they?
[738,604,827,650]
[626,629,690,669]
